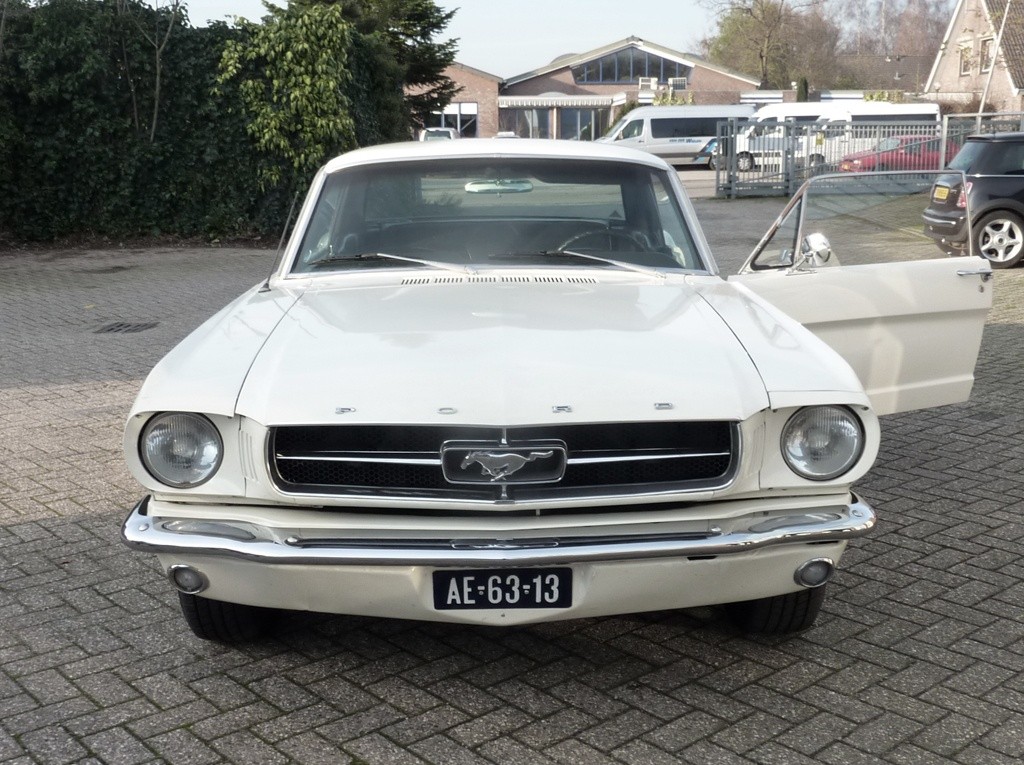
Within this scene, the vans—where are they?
[420,128,460,142]
[592,106,755,169]
[732,102,939,178]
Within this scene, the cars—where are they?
[121,137,994,646]
[492,131,519,138]
[839,135,958,176]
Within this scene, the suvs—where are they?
[921,133,1024,271]
